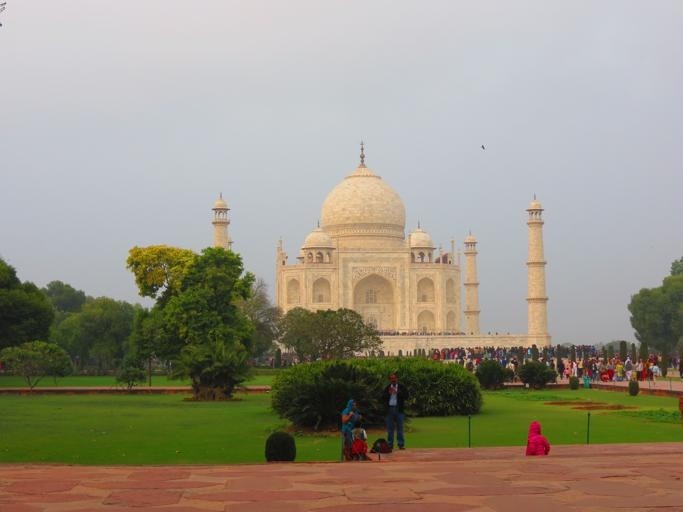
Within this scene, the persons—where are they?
[523,420,550,456]
[430,343,683,383]
[383,373,409,449]
[337,398,361,462]
[349,421,368,460]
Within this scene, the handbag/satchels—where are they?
[372,438,392,453]
